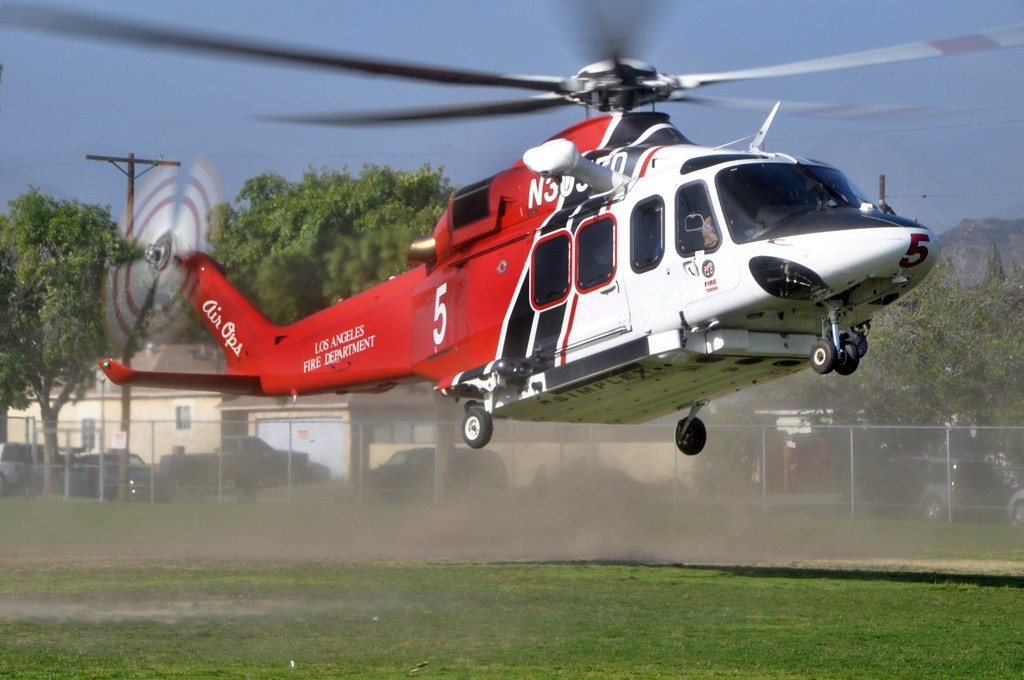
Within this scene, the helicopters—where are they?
[1,0,1024,455]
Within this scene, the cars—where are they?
[362,447,507,497]
[0,443,152,494]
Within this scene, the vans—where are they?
[893,460,1005,519]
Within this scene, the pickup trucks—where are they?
[158,434,310,487]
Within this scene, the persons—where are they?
[654,237,688,261]
[701,217,719,247]
